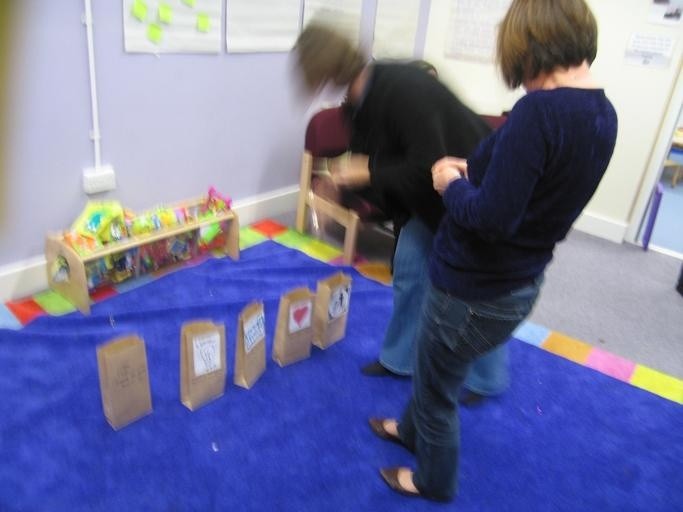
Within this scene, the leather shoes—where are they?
[379,467,422,496]
[362,362,390,376]
[370,415,403,444]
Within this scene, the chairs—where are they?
[295,107,391,268]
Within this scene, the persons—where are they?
[292,21,510,406]
[368,0,617,503]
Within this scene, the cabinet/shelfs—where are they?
[45,197,240,314]
